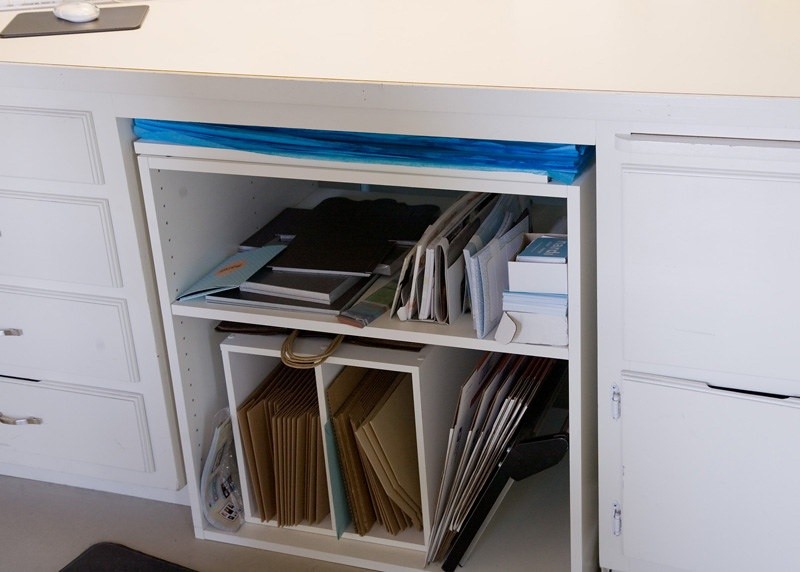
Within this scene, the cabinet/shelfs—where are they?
[0,96,800,572]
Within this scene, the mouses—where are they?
[54,3,100,23]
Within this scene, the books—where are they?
[172,183,570,347]
[200,416,245,533]
